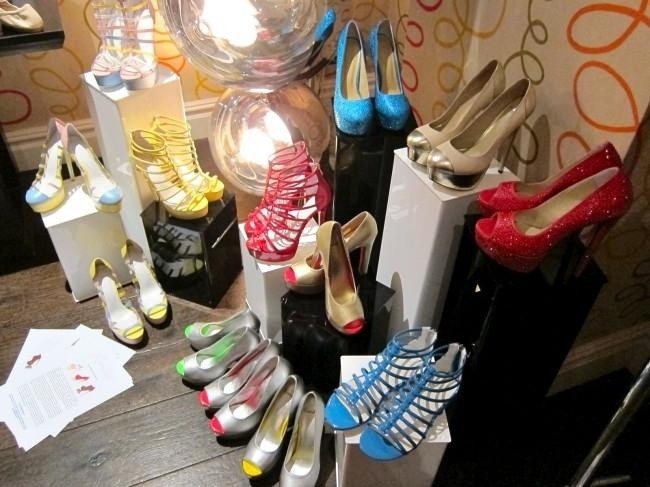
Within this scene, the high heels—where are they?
[25,116,75,212]
[148,114,224,202]
[474,165,634,277]
[427,77,537,191]
[309,220,365,333]
[129,128,209,220]
[245,140,315,239]
[478,140,624,216]
[242,372,325,487]
[283,210,378,293]
[246,162,332,261]
[61,123,124,213]
[407,59,506,166]
[199,338,293,439]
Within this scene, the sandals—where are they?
[90,256,145,345]
[121,238,168,324]
[175,307,261,384]
[325,326,468,461]
[91,0,157,90]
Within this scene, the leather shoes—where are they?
[367,18,410,130]
[333,18,374,134]
